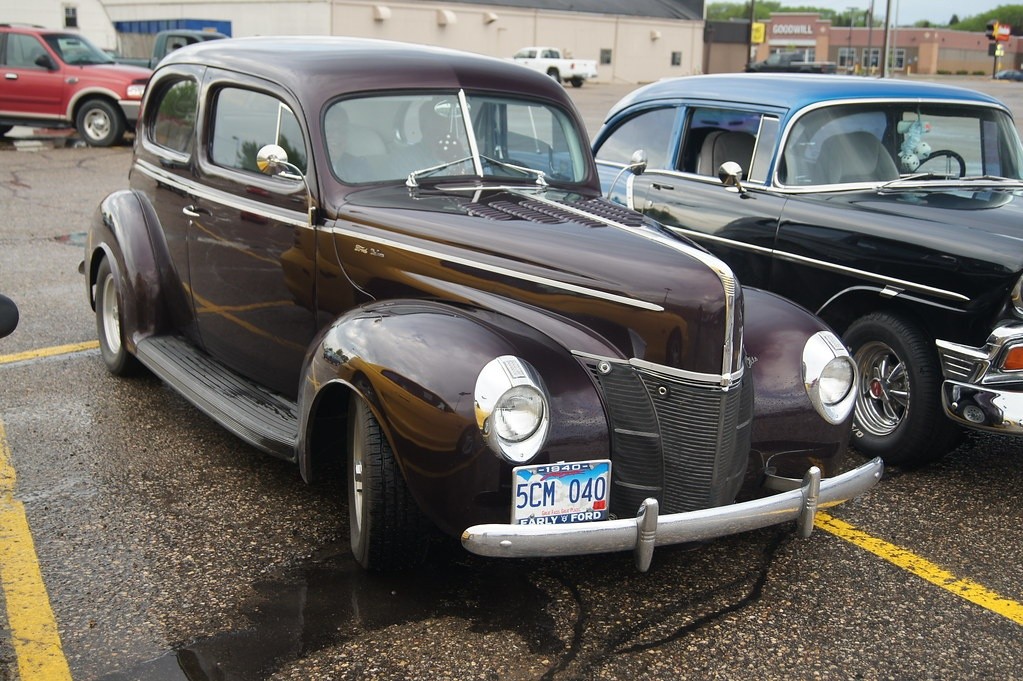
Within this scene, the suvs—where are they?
[0,21,177,148]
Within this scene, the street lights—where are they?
[846,6,859,67]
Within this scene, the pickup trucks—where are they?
[28,26,233,117]
[743,51,838,74]
[502,45,599,87]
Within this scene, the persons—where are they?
[324,104,375,181]
[391,100,494,179]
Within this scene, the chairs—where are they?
[694,130,757,185]
[810,130,900,184]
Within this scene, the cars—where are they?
[451,67,1023,472]
[77,31,887,584]
[989,69,1023,82]
